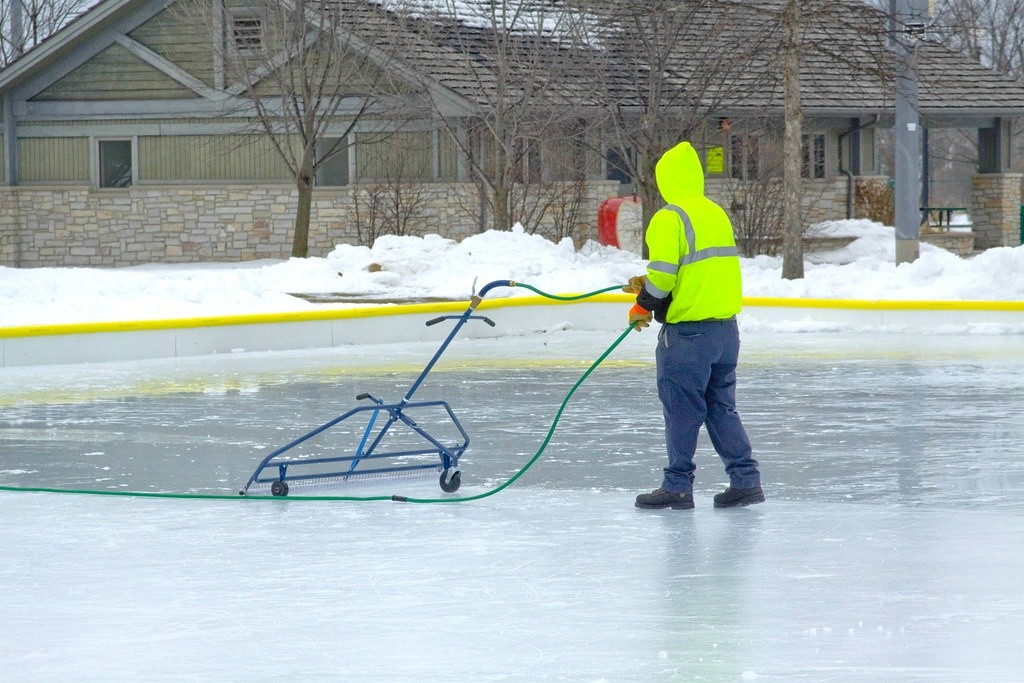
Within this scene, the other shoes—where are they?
[635,487,694,510]
[713,485,765,509]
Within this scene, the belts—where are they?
[701,315,738,321]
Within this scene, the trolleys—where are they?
[235,307,498,497]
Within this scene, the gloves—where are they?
[622,275,647,295]
[629,304,653,333]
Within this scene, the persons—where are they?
[622,141,765,510]
